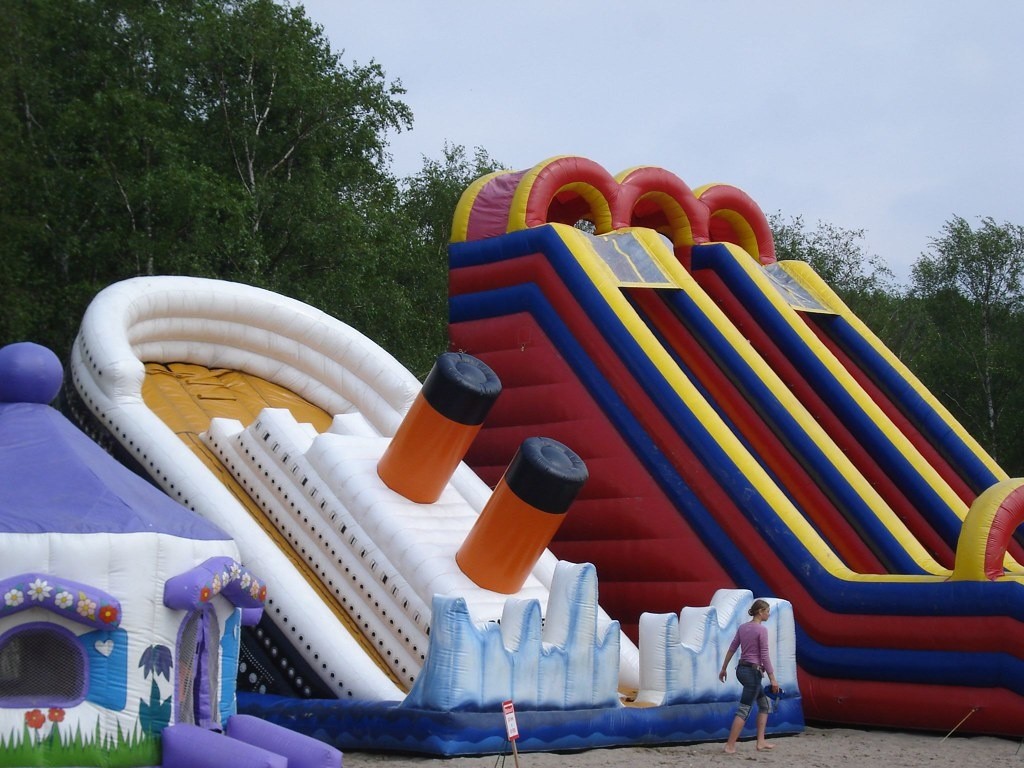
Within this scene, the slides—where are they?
[65,276,807,755]
[441,155,1023,739]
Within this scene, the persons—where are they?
[719,599,780,755]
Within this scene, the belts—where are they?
[738,660,766,680]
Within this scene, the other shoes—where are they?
[764,685,785,702]
